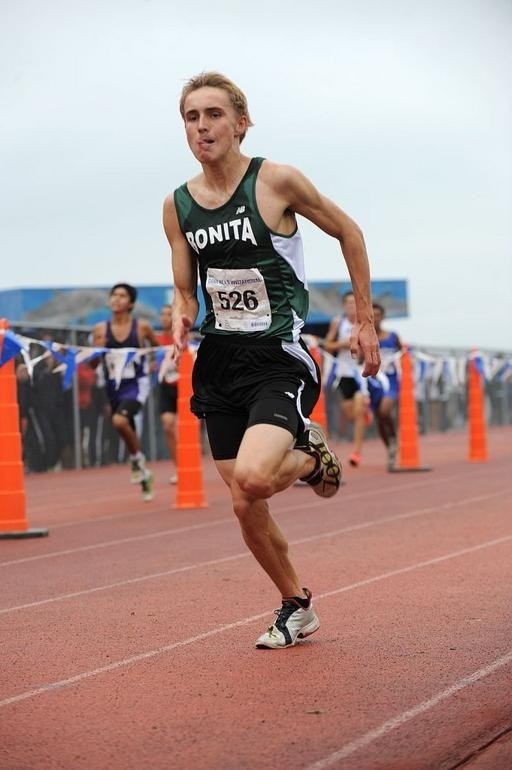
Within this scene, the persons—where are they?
[164,72,381,649]
[0,284,512,501]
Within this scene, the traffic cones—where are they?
[293,345,345,489]
[462,344,493,466]
[387,349,435,475]
[171,333,211,513]
[0,318,53,538]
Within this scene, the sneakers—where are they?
[295,422,343,498]
[129,454,146,484]
[348,452,362,467]
[142,470,154,503]
[254,587,320,650]
